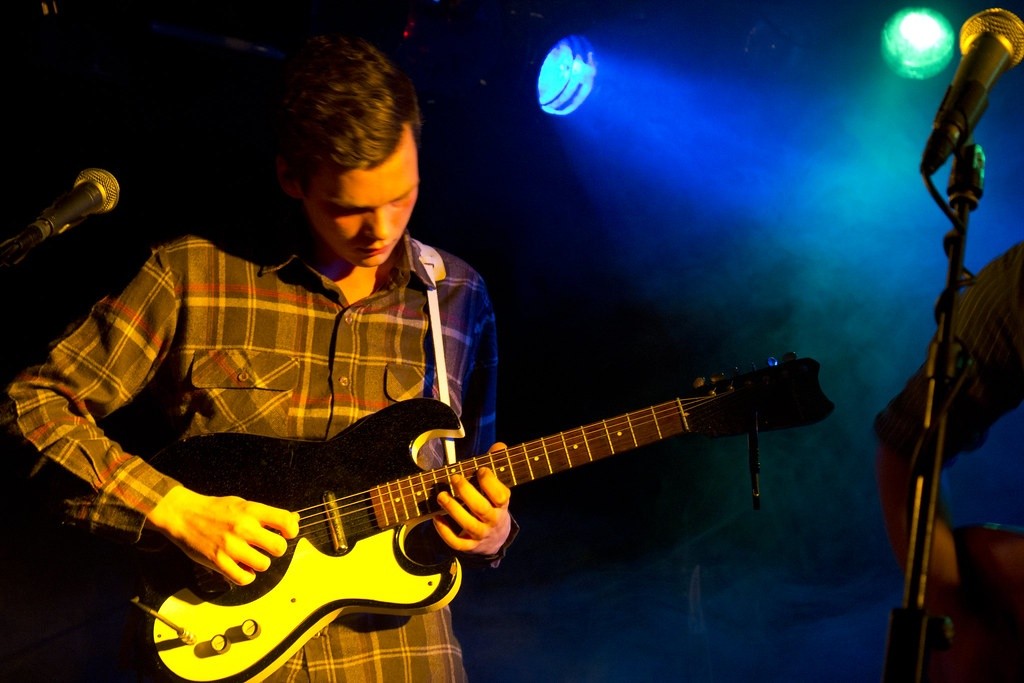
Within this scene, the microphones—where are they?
[920,8,1024,177]
[15,167,119,252]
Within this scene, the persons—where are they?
[0,35,519,683]
[873,241,1024,683]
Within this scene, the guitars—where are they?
[135,346,840,683]
[927,521,1023,682]
